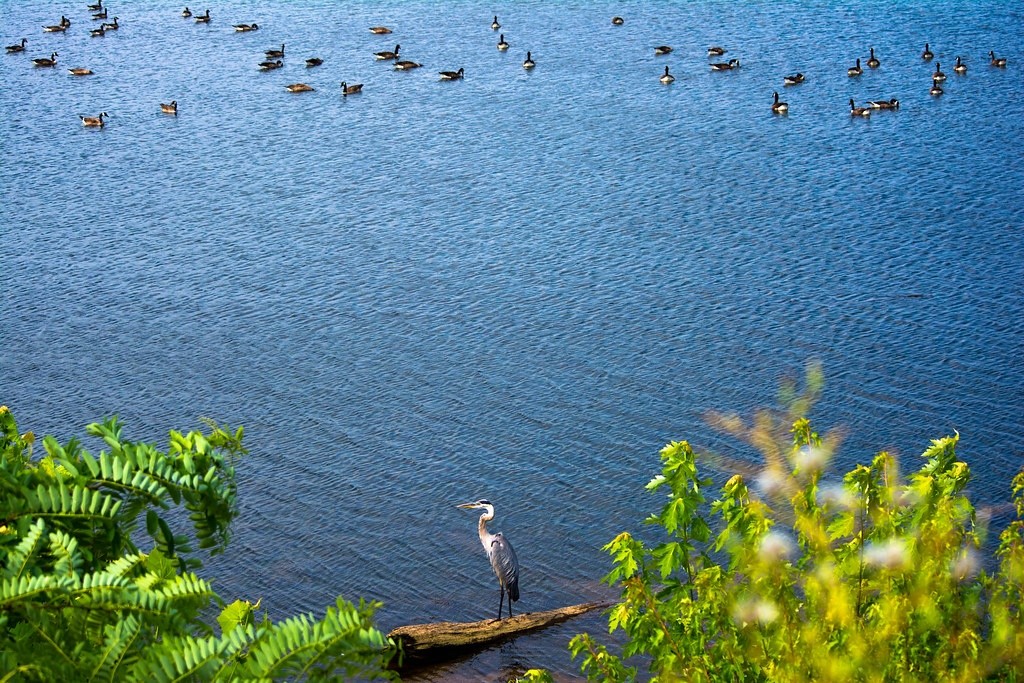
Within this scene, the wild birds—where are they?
[368,27,393,34]
[654,46,739,83]
[67,68,93,74]
[31,52,59,66]
[232,23,258,31]
[160,101,178,113]
[372,45,464,79]
[921,43,966,95]
[79,112,109,127]
[5,38,29,52]
[988,50,1007,65]
[340,81,364,95]
[492,16,535,69]
[40,0,120,36]
[182,7,211,21]
[455,499,519,619]
[257,44,324,91]
[770,47,899,116]
[612,17,624,25]
